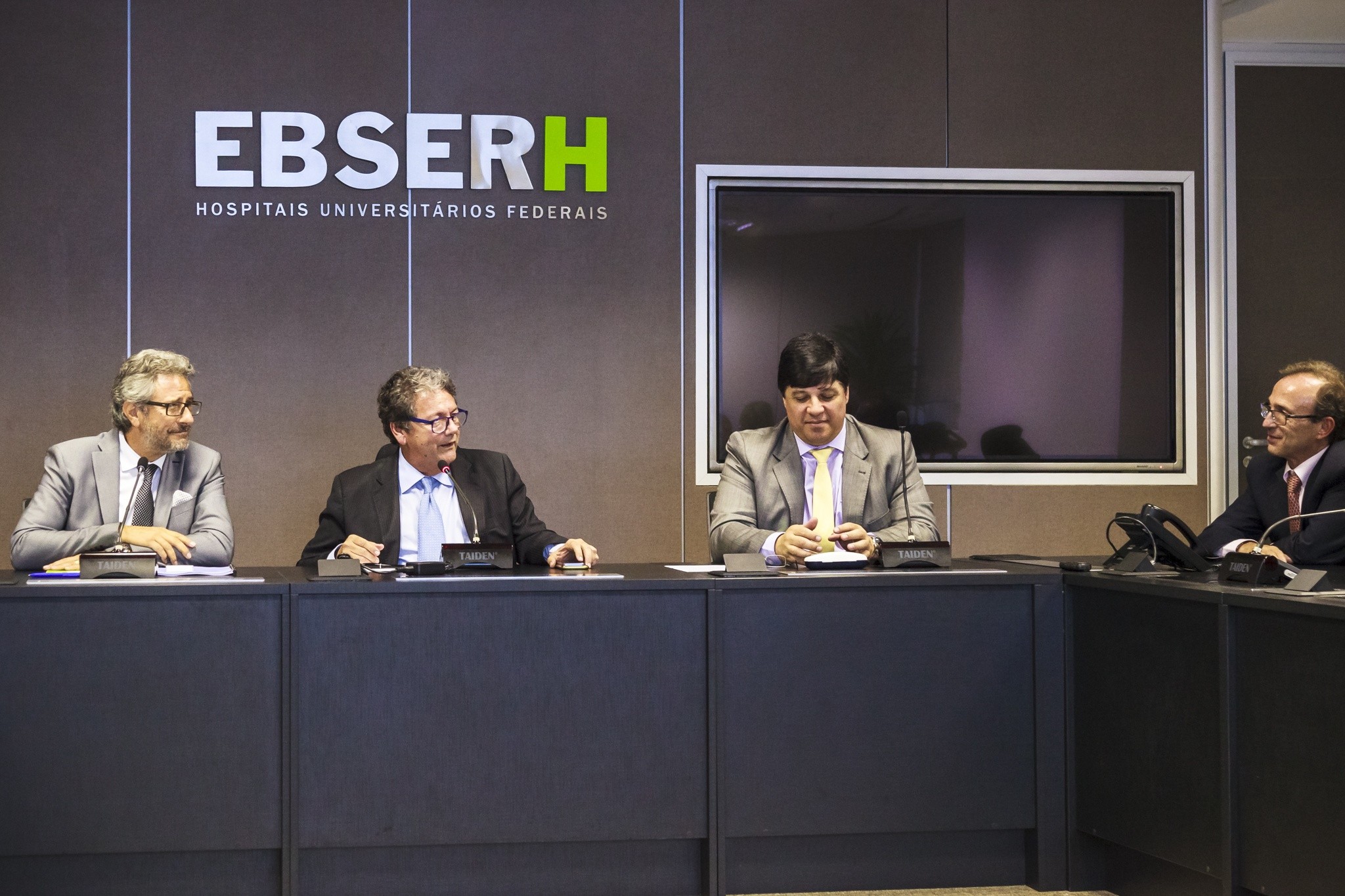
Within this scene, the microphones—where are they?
[79,456,157,578]
[438,460,515,570]
[869,410,952,567]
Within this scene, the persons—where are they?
[9,349,236,575]
[1195,358,1345,563]
[294,366,600,569]
[709,331,940,567]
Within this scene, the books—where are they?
[156,564,235,576]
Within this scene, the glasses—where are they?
[1259,401,1324,426]
[137,400,203,416]
[392,408,468,434]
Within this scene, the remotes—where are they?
[1059,561,1092,571]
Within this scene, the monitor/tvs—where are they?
[707,178,1187,470]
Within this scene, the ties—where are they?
[130,464,158,527]
[807,447,836,556]
[1286,472,1300,538]
[417,477,445,563]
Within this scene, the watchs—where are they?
[869,535,882,561]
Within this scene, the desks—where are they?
[0,555,1345,896]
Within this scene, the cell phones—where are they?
[557,559,588,570]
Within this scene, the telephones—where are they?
[1101,502,1210,572]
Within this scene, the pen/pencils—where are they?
[28,573,81,579]
